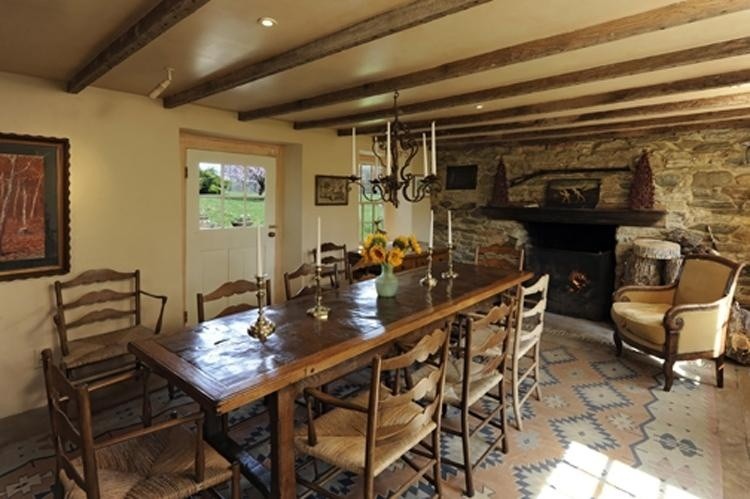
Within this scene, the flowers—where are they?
[361,232,422,267]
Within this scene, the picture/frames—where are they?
[0,132,71,282]
[315,175,348,206]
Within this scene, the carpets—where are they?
[0,313,722,498]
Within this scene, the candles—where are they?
[423,132,428,178]
[374,141,378,180]
[256,224,262,276]
[431,121,437,176]
[352,127,356,176]
[386,121,391,177]
[448,210,453,244]
[429,210,434,248]
[316,216,322,266]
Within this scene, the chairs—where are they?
[40,349,240,499]
[313,243,348,287]
[452,273,550,431]
[382,298,513,498]
[52,268,168,448]
[474,245,525,318]
[292,320,452,498]
[284,264,340,300]
[197,279,272,323]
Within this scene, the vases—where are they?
[375,265,399,297]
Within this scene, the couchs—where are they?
[611,255,746,391]
